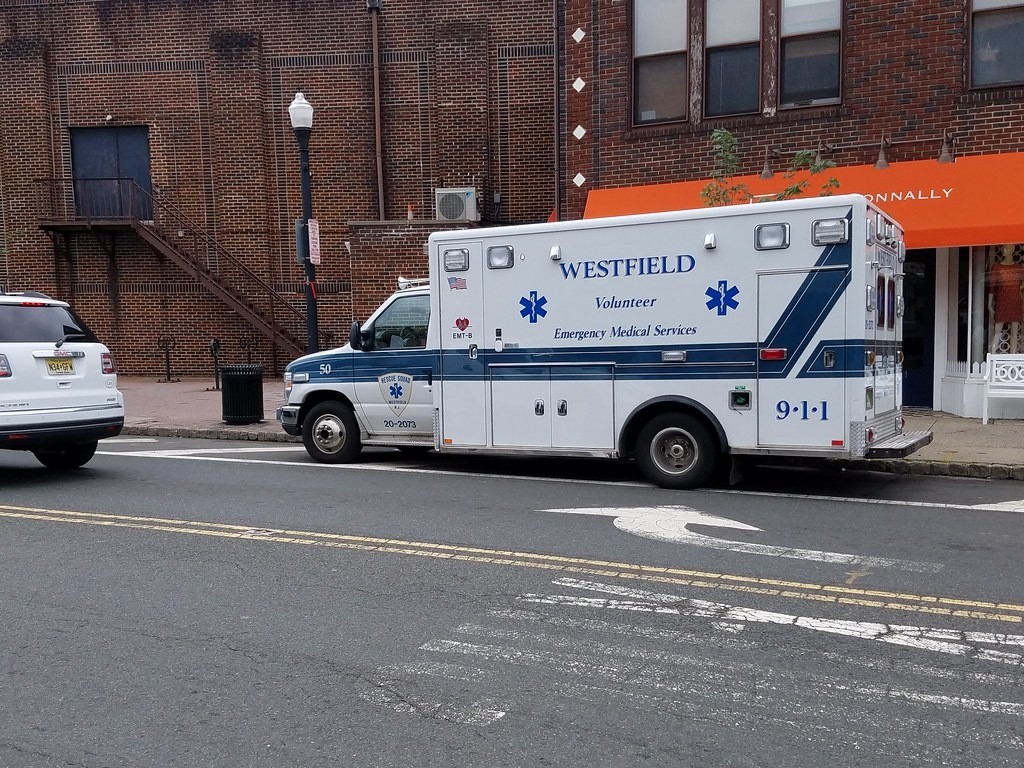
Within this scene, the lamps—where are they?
[815,139,834,170]
[759,146,781,178]
[874,134,892,168]
[937,128,954,161]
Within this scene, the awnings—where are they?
[584,152,1024,250]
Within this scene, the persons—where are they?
[988,244,1024,354]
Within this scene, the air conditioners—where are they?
[435,187,480,221]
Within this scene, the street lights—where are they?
[287,91,319,358]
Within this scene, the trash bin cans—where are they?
[215,363,268,423]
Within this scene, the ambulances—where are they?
[274,191,936,490]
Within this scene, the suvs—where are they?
[0,291,126,473]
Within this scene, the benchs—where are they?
[982,353,1024,424]
